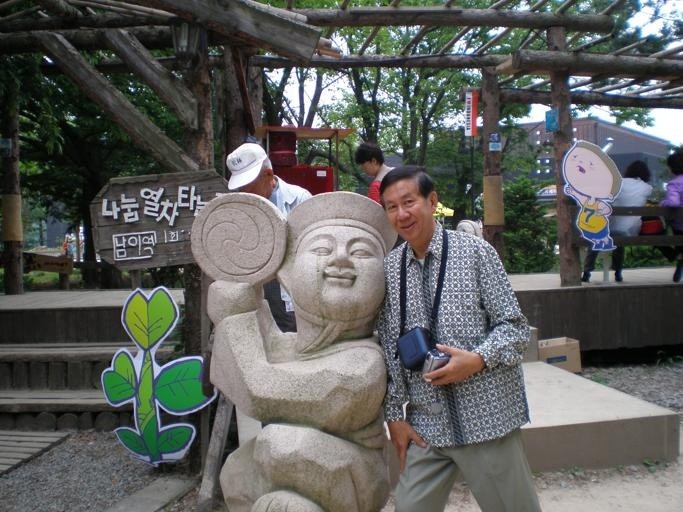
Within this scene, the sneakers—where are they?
[603,238,613,249]
[615,273,622,282]
[581,272,591,283]
[673,259,683,282]
[592,240,604,251]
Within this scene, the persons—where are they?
[658,151,683,284]
[377,165,541,511]
[206,192,393,512]
[356,143,397,202]
[581,158,652,284]
[226,144,312,332]
[63,227,73,258]
[563,140,624,251]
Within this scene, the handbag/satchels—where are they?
[639,215,666,235]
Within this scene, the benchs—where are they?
[577,205,682,283]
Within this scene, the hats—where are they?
[226,143,267,189]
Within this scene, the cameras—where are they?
[421,347,450,379]
